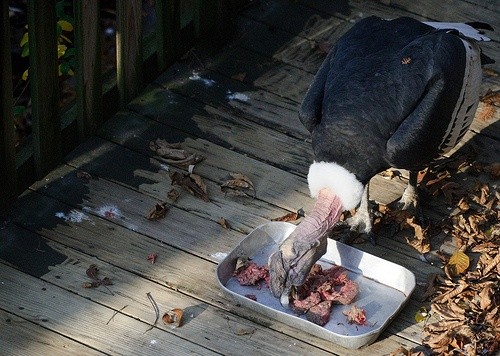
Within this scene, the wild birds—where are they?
[268,14,495,310]
